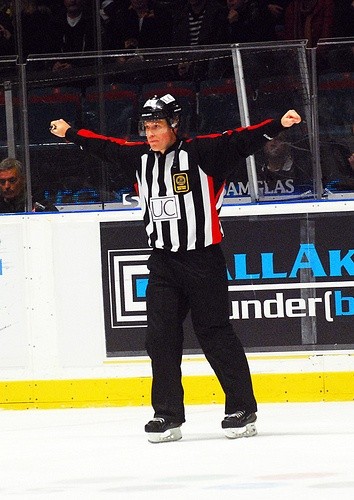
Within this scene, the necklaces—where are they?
[265,152,290,170]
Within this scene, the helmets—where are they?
[138,94,184,136]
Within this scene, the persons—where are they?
[49,93,302,443]
[0,0,354,89]
[245,130,313,196]
[348,154,354,173]
[0,158,59,213]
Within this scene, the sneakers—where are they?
[144,415,182,442]
[221,410,257,439]
